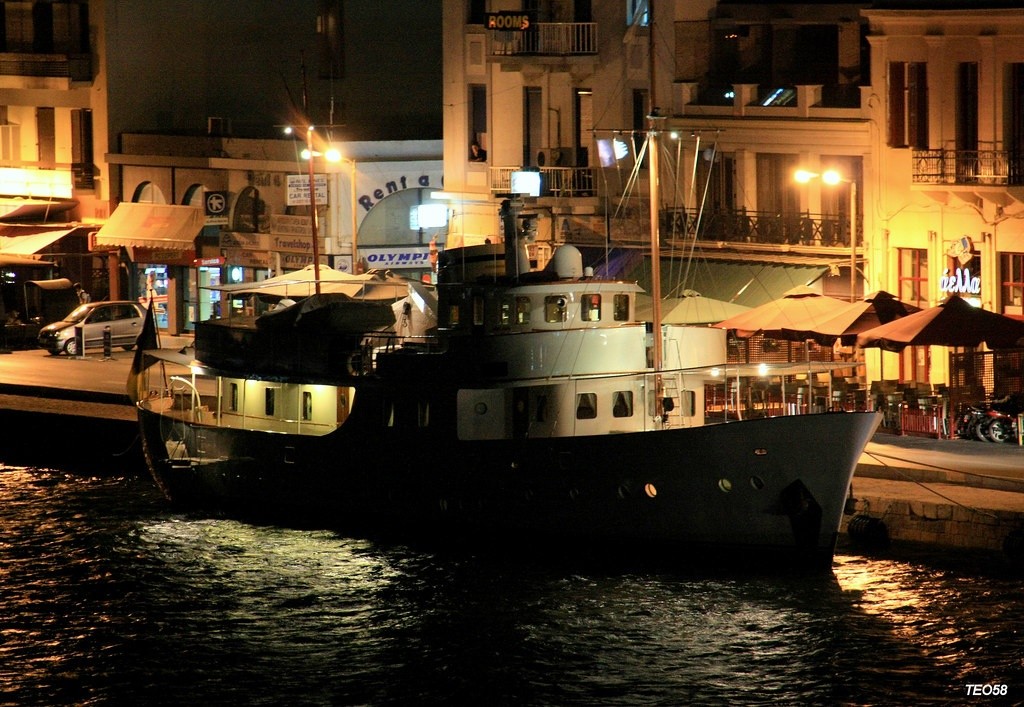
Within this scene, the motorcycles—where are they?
[953,392,1024,443]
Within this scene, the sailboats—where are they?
[137,0,885,583]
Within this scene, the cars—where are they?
[38,301,147,356]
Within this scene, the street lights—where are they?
[284,125,346,294]
[791,162,858,301]
